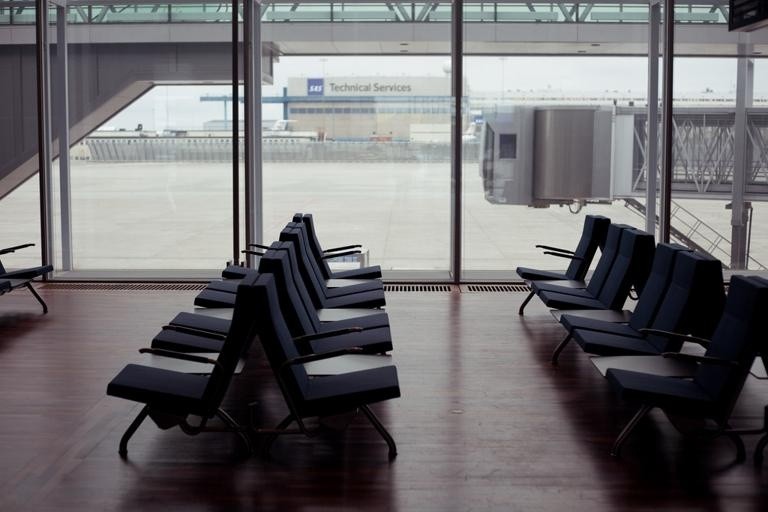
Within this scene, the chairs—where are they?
[106,269,260,461]
[252,273,401,461]
[0,243,54,313]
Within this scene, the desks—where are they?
[0,277,32,291]
